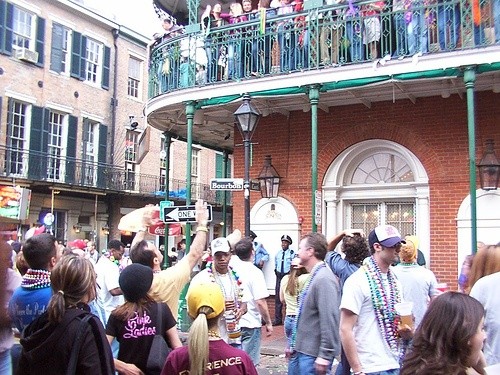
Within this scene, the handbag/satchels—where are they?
[145,303,173,373]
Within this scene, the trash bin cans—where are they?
[176,283,191,330]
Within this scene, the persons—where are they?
[394,233,439,331]
[234,239,272,369]
[161,281,258,375]
[86,240,101,264]
[327,230,370,294]
[278,256,312,361]
[457,255,474,295]
[186,237,248,350]
[337,225,417,375]
[241,0,255,79]
[471,244,500,375]
[10,233,64,330]
[269,0,499,80]
[399,291,489,375]
[259,1,268,78]
[274,236,297,326]
[284,232,338,375]
[178,239,189,254]
[95,239,124,310]
[203,1,222,86]
[251,231,269,273]
[130,197,209,332]
[12,257,114,374]
[220,3,246,79]
[158,17,182,92]
[104,262,184,375]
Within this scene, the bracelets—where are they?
[197,226,209,233]
[349,366,367,375]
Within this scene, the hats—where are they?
[226,229,241,252]
[211,237,231,256]
[187,281,225,319]
[119,264,153,301]
[250,230,257,239]
[69,240,87,249]
[25,225,45,240]
[399,235,419,261]
[281,235,292,244]
[369,224,407,248]
[291,257,304,268]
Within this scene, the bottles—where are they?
[225,312,241,338]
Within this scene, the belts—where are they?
[288,315,296,318]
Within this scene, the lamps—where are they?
[476,138,499,190]
[255,154,282,198]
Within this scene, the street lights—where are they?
[233,92,261,242]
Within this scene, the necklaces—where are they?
[205,261,243,327]
[360,255,407,364]
[396,262,418,266]
[209,331,221,338]
[20,268,52,290]
[102,249,124,271]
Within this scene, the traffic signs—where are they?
[250,179,260,191]
[163,205,213,226]
[209,177,244,193]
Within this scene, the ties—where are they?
[281,252,284,276]
